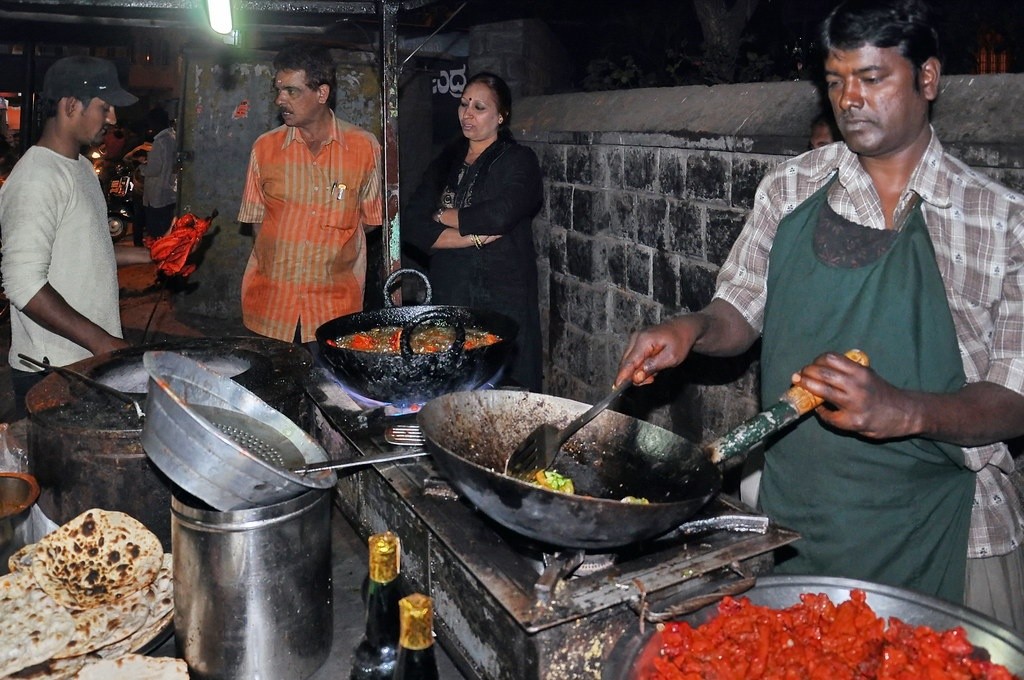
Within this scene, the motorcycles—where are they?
[86,145,136,241]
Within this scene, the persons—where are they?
[238,43,383,356]
[807,112,845,152]
[137,106,178,298]
[401,73,545,394]
[616,0,1024,629]
[0,56,139,409]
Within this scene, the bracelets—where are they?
[469,233,484,250]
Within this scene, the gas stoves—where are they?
[296,351,800,680]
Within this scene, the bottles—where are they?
[347,532,408,680]
[392,594,440,680]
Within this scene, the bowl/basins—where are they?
[137,349,338,504]
[0,471,40,531]
[598,574,1024,680]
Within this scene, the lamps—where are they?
[207,0,232,35]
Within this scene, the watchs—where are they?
[436,208,446,224]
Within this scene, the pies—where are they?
[0,508,192,680]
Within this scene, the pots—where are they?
[416,347,871,550]
[86,344,261,402]
[314,266,519,404]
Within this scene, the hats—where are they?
[43,56,139,107]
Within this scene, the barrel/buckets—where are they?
[171,486,334,679]
